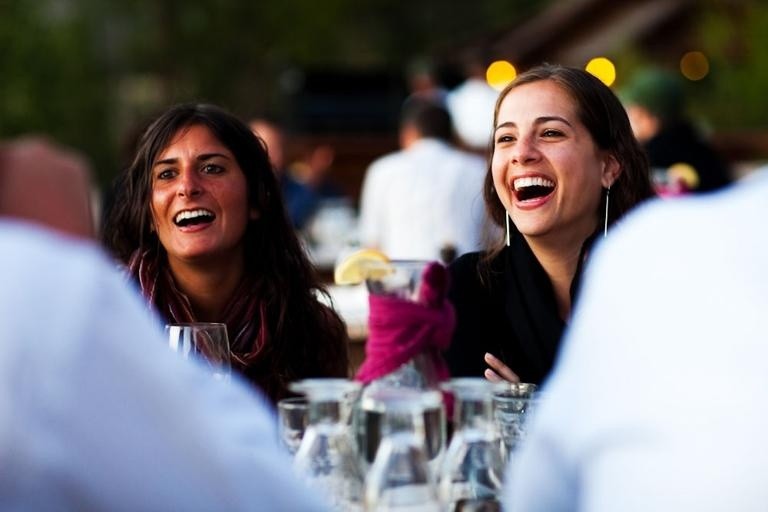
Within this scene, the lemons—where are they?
[334,249,395,286]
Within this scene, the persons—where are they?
[102,98,349,418]
[1,134,341,509]
[493,160,766,511]
[435,62,681,386]
[250,31,735,343]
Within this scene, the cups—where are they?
[363,259,432,302]
[163,319,233,379]
[275,365,542,512]
